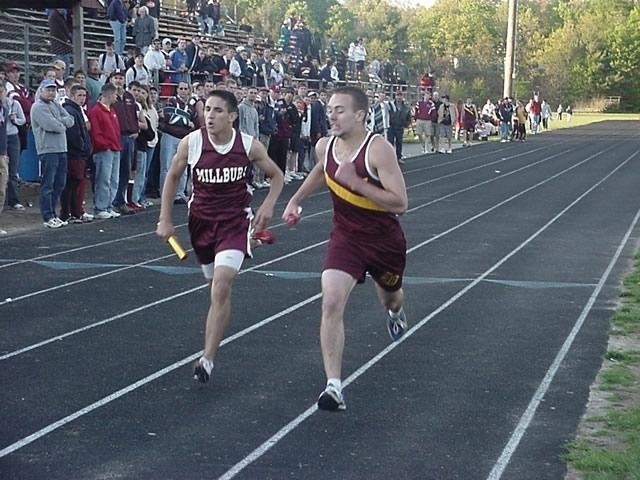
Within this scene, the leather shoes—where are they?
[127,201,145,212]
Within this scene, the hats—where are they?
[112,67,126,76]
[51,60,67,70]
[5,63,22,73]
[441,94,450,100]
[39,78,58,88]
[148,84,158,91]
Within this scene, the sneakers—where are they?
[141,199,154,207]
[119,204,138,215]
[435,149,438,153]
[13,204,25,210]
[251,180,263,189]
[447,148,452,154]
[94,210,111,220]
[66,215,83,225]
[261,181,271,188]
[440,148,445,153]
[79,213,95,222]
[109,210,121,218]
[195,356,216,384]
[43,219,61,229]
[431,147,435,153]
[56,216,69,226]
[317,384,346,413]
[385,306,409,342]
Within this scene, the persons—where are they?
[508,97,519,136]
[224,48,241,85]
[127,54,151,97]
[185,1,228,38]
[47,8,76,76]
[156,91,283,383]
[514,101,529,142]
[59,87,94,225]
[558,104,562,118]
[174,41,191,83]
[461,97,477,144]
[125,81,152,210]
[437,95,459,152]
[389,92,412,163]
[258,50,272,86]
[5,63,31,155]
[189,35,201,69]
[202,45,216,82]
[253,88,276,189]
[347,41,358,76]
[104,70,140,212]
[393,58,408,84]
[356,39,367,75]
[539,99,552,130]
[370,57,379,81]
[175,80,247,186]
[529,94,540,131]
[279,20,291,54]
[133,7,156,55]
[86,61,104,99]
[367,57,381,85]
[318,60,333,91]
[311,90,330,148]
[97,42,127,82]
[159,37,172,61]
[382,58,394,87]
[142,39,165,87]
[270,53,284,85]
[566,105,572,119]
[282,95,306,183]
[497,97,509,142]
[370,90,389,139]
[146,87,165,174]
[212,45,229,86]
[469,111,499,141]
[415,92,435,150]
[34,58,89,114]
[285,12,342,86]
[163,83,199,203]
[483,100,497,122]
[455,97,464,139]
[238,51,251,86]
[0,83,26,210]
[89,83,125,219]
[237,87,259,186]
[31,81,74,228]
[108,0,131,54]
[426,92,445,151]
[281,87,409,410]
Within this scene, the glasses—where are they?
[179,87,188,90]
[248,91,256,94]
[132,89,142,92]
